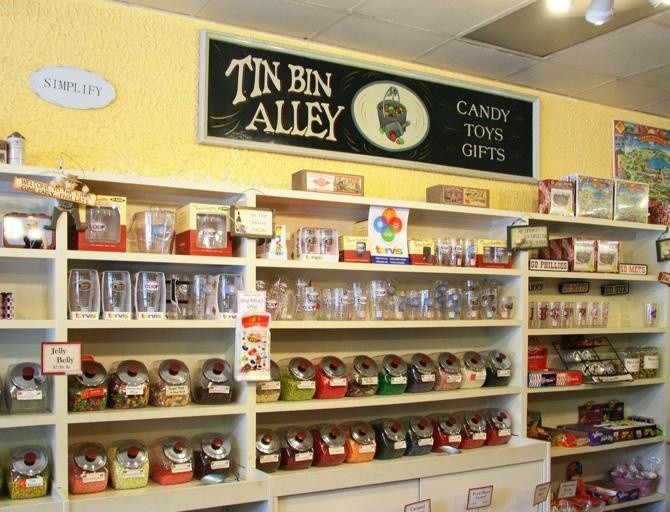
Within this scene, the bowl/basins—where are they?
[608,470,657,497]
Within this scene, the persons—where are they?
[44,174,90,232]
[22,215,48,250]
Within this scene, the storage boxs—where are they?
[292,169,364,198]
[427,184,489,208]
[538,174,653,228]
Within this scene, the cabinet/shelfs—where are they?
[246,186,523,474]
[278,459,546,512]
[526,211,670,510]
[0,164,252,512]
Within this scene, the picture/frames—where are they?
[610,117,670,224]
[197,26,539,186]
[507,224,550,251]
[228,205,276,241]
[655,237,670,262]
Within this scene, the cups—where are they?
[86,205,120,245]
[434,238,477,266]
[302,230,337,253]
[133,210,174,255]
[72,270,242,313]
[357,241,366,259]
[195,214,223,248]
[484,247,505,263]
[423,246,431,261]
[642,302,657,327]
[528,300,609,328]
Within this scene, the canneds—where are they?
[611,347,659,379]
[0,292,14,319]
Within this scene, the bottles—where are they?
[256,277,515,320]
[8,131,25,165]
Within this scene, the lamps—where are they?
[583,2,614,28]
[649,0,661,9]
